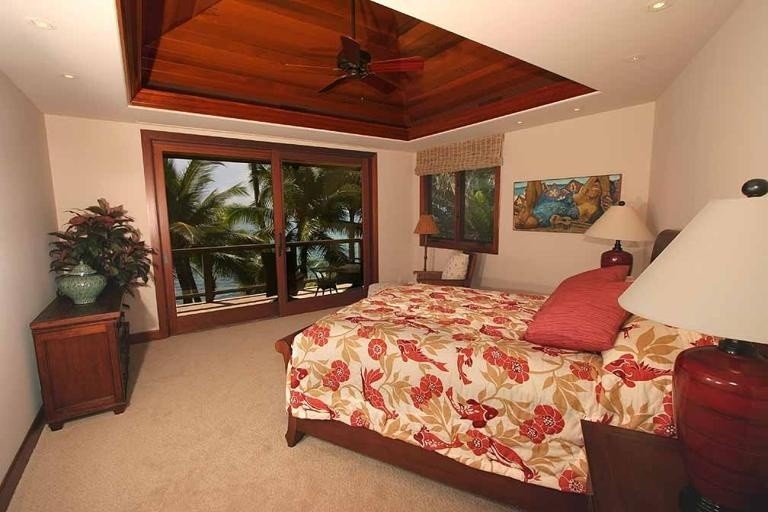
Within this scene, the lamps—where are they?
[413,215,440,271]
[619,179,768,512]
[583,200,654,276]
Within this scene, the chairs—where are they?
[413,252,478,288]
[261,251,304,302]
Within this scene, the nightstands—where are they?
[581,419,688,512]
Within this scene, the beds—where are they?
[275,231,726,512]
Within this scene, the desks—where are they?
[310,267,343,296]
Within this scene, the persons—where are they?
[513,174,622,231]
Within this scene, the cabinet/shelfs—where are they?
[30,289,130,432]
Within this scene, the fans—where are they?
[281,0,424,97]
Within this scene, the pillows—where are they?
[442,254,470,281]
[523,281,635,352]
[532,265,630,321]
